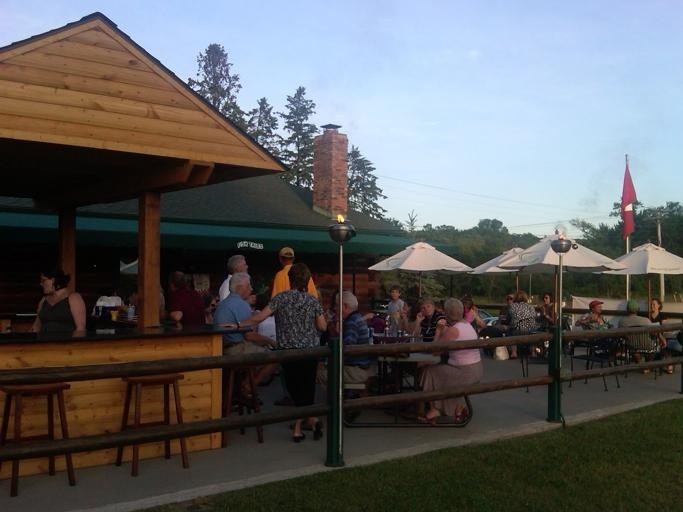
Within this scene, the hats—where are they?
[589,301,603,307]
[280,247,294,257]
[627,300,639,311]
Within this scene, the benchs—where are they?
[343,380,480,427]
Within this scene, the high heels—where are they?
[455,406,469,424]
[313,421,323,439]
[417,413,439,424]
[293,433,305,442]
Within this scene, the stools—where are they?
[115,373,189,476]
[1,383,76,497]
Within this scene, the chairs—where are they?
[516,316,573,394]
[580,322,620,391]
[625,324,660,379]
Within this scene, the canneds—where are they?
[368,327,375,337]
[384,329,389,337]
[397,330,402,338]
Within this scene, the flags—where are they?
[621,162,637,241]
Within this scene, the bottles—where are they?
[366,325,426,346]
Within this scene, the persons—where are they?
[92,286,138,315]
[30,265,86,332]
[159,243,485,444]
[495,292,559,359]
[575,301,674,375]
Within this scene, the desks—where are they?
[374,332,423,337]
[378,352,442,423]
